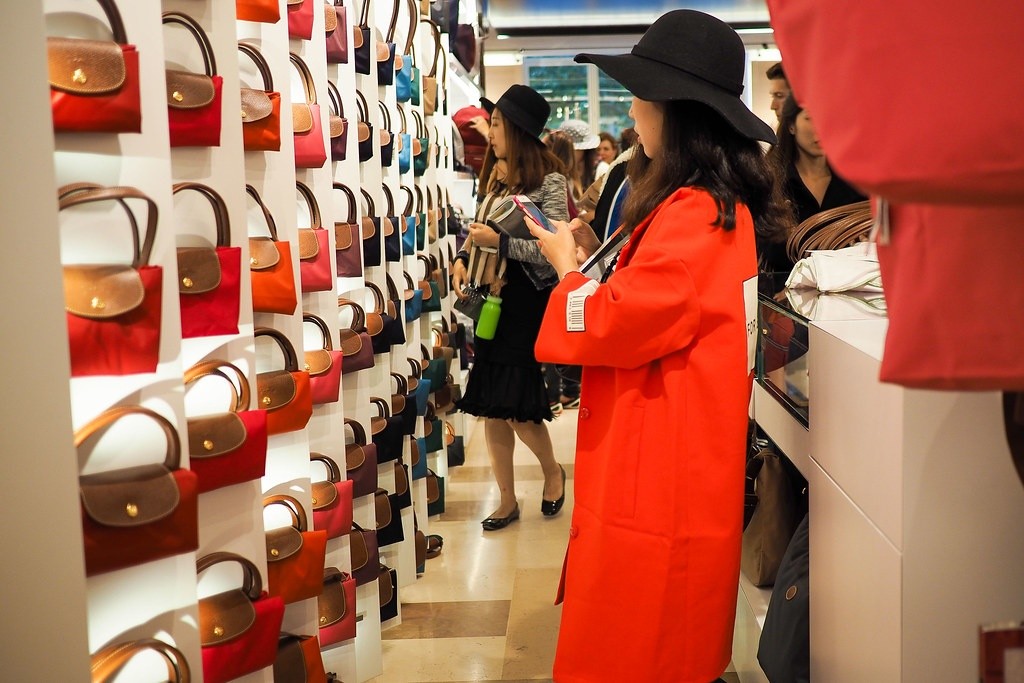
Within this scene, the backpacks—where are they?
[452,104,491,168]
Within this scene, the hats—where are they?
[559,120,601,152]
[573,9,779,144]
[479,84,547,146]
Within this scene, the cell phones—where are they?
[513,195,558,233]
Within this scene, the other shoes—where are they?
[540,464,566,516]
[481,501,521,530]
[549,395,580,414]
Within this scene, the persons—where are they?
[523,9,798,683]
[755,61,874,297]
[452,83,639,531]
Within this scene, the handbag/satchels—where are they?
[739,436,808,683]
[42,0,465,683]
[453,287,486,321]
